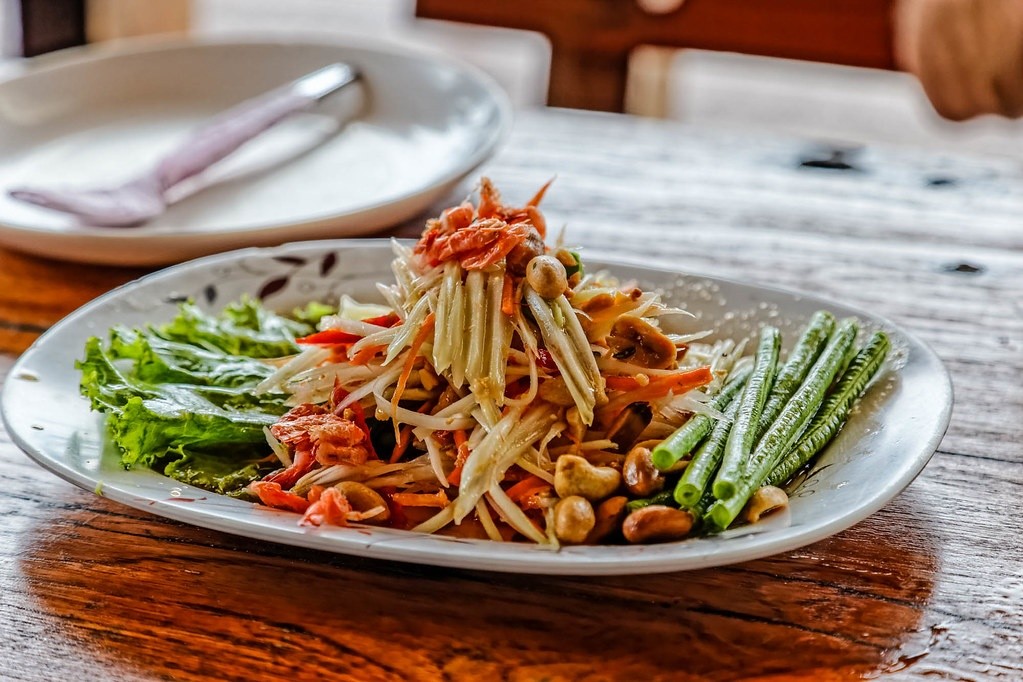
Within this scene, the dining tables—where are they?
[0,108,1021,682]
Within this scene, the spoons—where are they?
[10,58,359,228]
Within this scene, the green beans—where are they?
[636,309,889,524]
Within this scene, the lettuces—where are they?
[76,293,332,489]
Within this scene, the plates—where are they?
[0,25,512,266]
[1,234,959,577]
